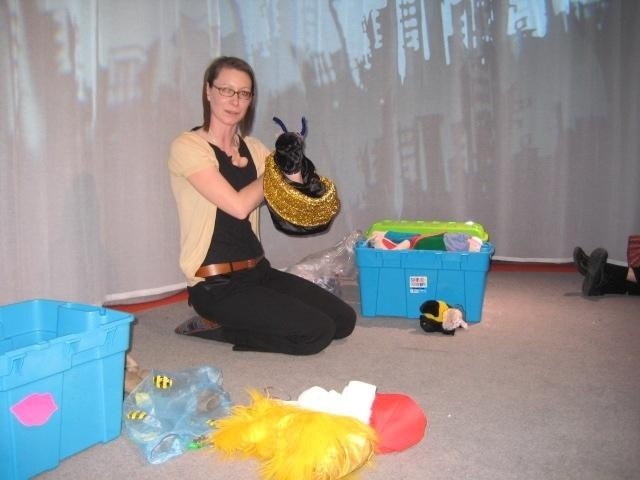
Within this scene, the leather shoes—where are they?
[573,247,607,296]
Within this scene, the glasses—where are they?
[211,84,252,100]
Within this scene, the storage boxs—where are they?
[364,219,488,241]
[354,240,496,324]
[0,298,135,480]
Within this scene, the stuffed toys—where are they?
[365,230,481,252]
[263,114,340,237]
[419,299,469,336]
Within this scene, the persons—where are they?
[572,236,639,298]
[166,56,357,356]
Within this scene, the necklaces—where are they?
[208,131,238,161]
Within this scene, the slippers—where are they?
[174,316,222,334]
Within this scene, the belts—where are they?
[195,254,264,278]
